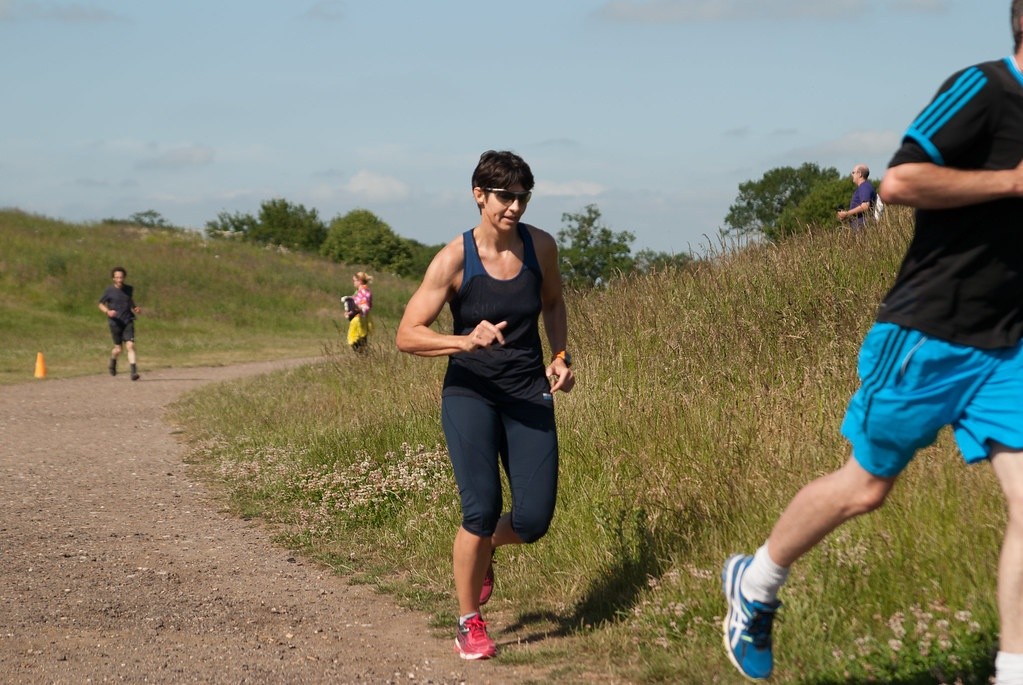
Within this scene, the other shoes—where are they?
[109,365,117,375]
[132,374,140,380]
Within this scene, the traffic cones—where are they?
[34,352,47,379]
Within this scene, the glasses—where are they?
[484,188,532,205]
[852,171,858,174]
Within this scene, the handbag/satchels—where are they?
[875,194,883,221]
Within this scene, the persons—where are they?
[395,149,575,660]
[835,164,877,229]
[98,267,142,380]
[341,271,373,356]
[722,0,1023,685]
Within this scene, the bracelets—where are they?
[106,309,109,315]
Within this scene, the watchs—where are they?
[551,350,572,368]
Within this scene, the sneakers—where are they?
[478,552,497,605]
[453,614,496,659]
[722,555,781,684]
